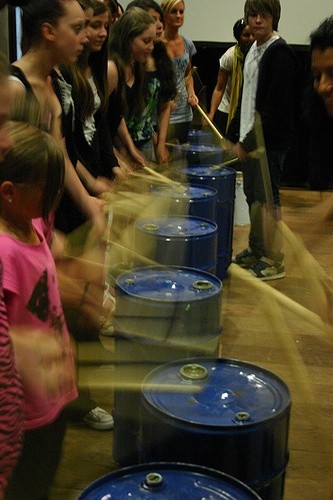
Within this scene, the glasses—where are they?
[236,19,248,30]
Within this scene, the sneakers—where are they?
[83,405,115,430]
[98,282,116,336]
[232,246,285,280]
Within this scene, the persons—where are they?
[0,0,256,500]
[309,15,333,121]
[222,0,295,280]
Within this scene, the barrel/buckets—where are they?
[74,462,263,500]
[112,264,223,466]
[134,215,218,277]
[175,165,237,274]
[172,142,225,166]
[149,182,218,224]
[187,123,213,143]
[137,357,292,500]
